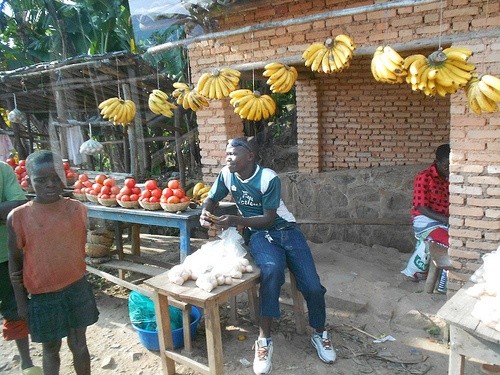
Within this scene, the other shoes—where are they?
[18,362,44,375]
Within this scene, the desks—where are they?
[80,200,238,266]
[143,259,305,375]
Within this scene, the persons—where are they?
[7,149,101,375]
[0,161,43,375]
[199,137,337,375]
[409,144,450,292]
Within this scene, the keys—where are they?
[265,234,273,243]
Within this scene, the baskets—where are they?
[72,193,190,212]
[84,228,115,258]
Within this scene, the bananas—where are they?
[196,68,241,99]
[263,63,298,93]
[193,182,211,206]
[98,97,136,126]
[371,46,477,97]
[463,71,500,116]
[172,83,210,112]
[229,89,275,121]
[149,89,177,118]
[302,34,356,74]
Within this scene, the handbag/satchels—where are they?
[400,241,431,281]
[127,290,183,331]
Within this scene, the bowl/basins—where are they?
[72,193,89,202]
[97,198,118,207]
[86,193,98,203]
[138,200,161,211]
[66,178,78,186]
[132,306,201,350]
[85,230,113,258]
[25,185,35,193]
[160,201,190,212]
[116,199,139,208]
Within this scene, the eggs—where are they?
[168,258,253,291]
[80,144,104,155]
[8,112,26,123]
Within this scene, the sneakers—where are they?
[253,336,273,375]
[310,330,336,364]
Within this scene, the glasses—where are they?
[226,139,252,153]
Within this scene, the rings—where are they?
[222,228,223,230]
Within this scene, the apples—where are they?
[5,158,29,189]
[74,174,190,204]
[62,162,79,181]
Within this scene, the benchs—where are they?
[424,239,448,295]
